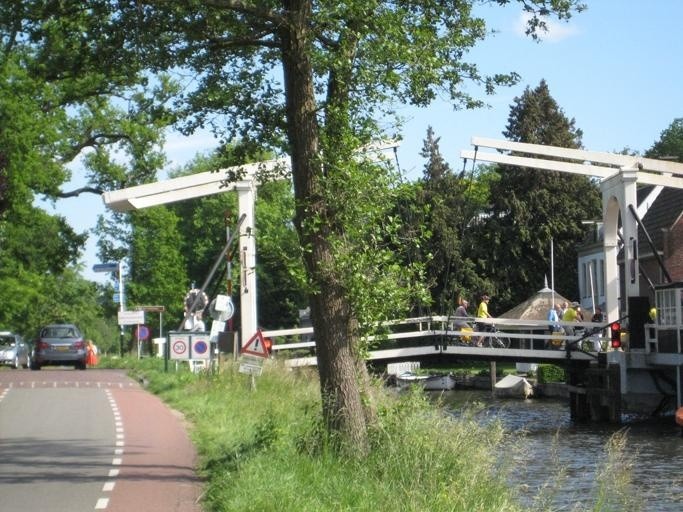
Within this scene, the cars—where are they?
[0,333,31,370]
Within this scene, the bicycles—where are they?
[546,323,601,353]
[451,323,511,350]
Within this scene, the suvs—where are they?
[30,323,87,369]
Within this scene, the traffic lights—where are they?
[609,322,621,348]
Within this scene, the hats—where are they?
[572,302,580,307]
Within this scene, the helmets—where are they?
[482,296,490,300]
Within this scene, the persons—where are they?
[476,294,492,347]
[455,300,474,343]
[548,302,604,352]
[86,340,97,369]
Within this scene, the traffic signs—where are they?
[168,332,188,360]
[92,263,118,272]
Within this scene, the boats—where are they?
[492,372,534,400]
[395,370,454,393]
